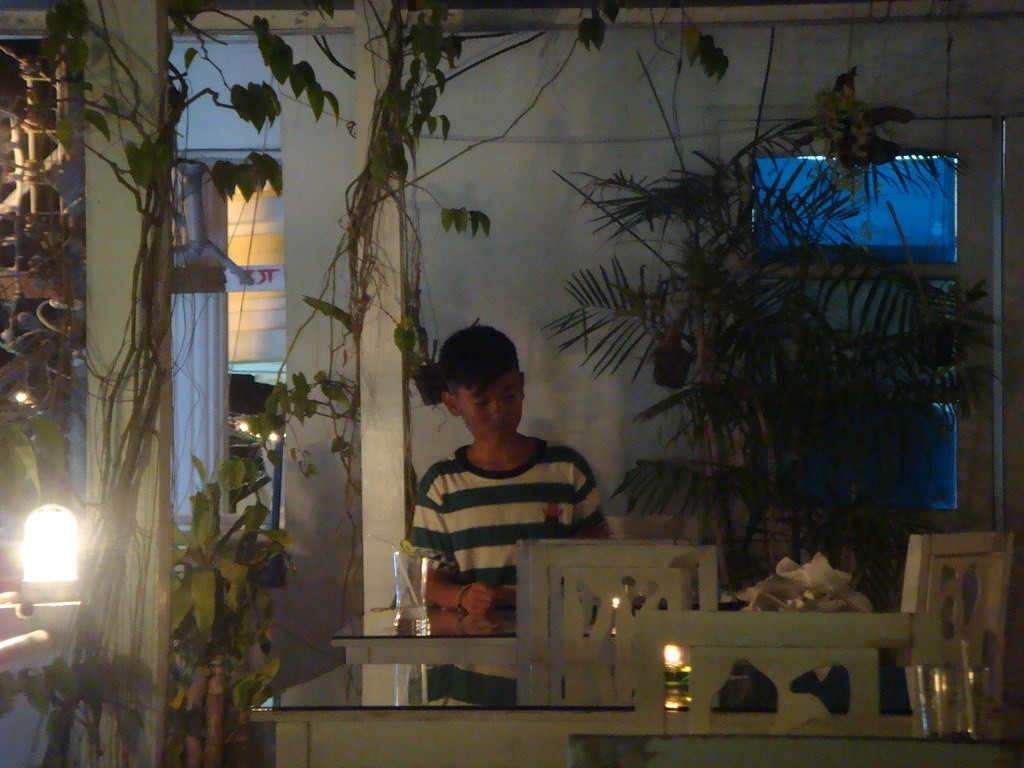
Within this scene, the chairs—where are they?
[515,532,1014,734]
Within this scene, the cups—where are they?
[392,552,429,620]
[919,665,994,741]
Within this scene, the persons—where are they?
[424,607,497,636]
[407,324,610,707]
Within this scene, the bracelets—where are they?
[457,613,468,623]
[455,584,471,612]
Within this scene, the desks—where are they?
[330,599,945,697]
[253,665,1024,768]
